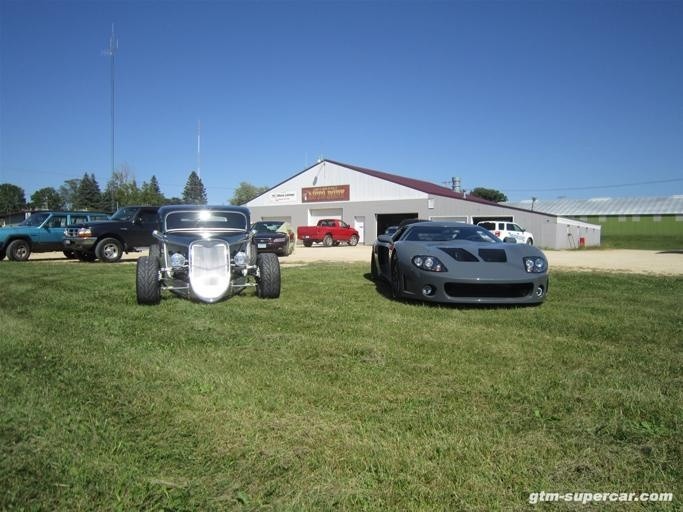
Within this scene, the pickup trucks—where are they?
[298,219,360,247]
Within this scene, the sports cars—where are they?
[371,222,550,303]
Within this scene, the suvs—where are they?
[0,206,160,262]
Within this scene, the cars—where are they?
[136,204,296,305]
[385,219,430,236]
[477,220,535,245]
